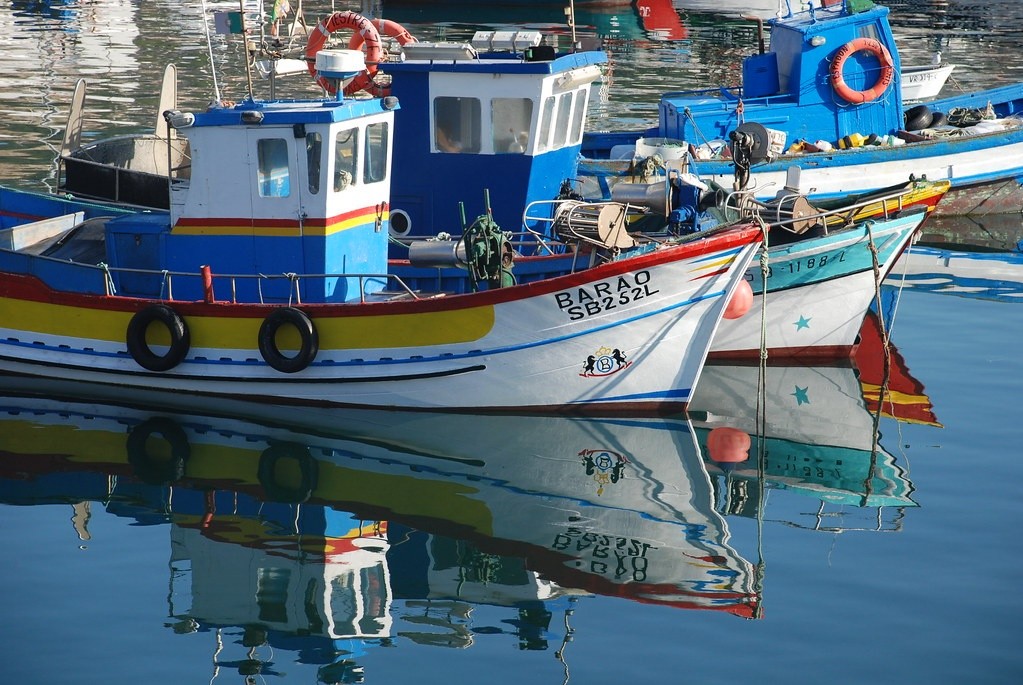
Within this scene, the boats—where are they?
[900,51,956,106]
[361,0,687,46]
[57,63,952,363]
[1,49,773,409]
[1,369,766,664]
[580,1,1023,216]
[689,364,919,533]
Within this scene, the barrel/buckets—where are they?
[610,144,636,159]
[634,137,689,173]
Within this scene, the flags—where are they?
[271,0,290,20]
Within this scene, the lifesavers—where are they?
[348,17,414,98]
[125,304,191,372]
[258,306,320,373]
[257,445,319,504]
[307,9,382,96]
[905,105,949,132]
[125,417,192,486]
[831,36,893,104]
